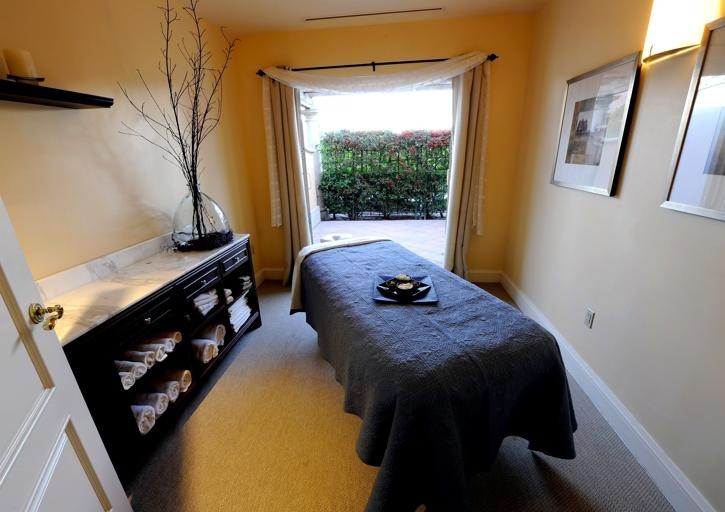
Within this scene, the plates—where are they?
[372,273,440,303]
[376,276,431,301]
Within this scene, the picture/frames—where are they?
[658,16,725,221]
[549,50,642,196]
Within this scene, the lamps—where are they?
[642,0,722,63]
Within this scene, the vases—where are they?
[171,183,234,252]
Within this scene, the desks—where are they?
[289,237,579,512]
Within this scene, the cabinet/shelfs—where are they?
[62,239,263,498]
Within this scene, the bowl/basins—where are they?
[396,280,419,294]
